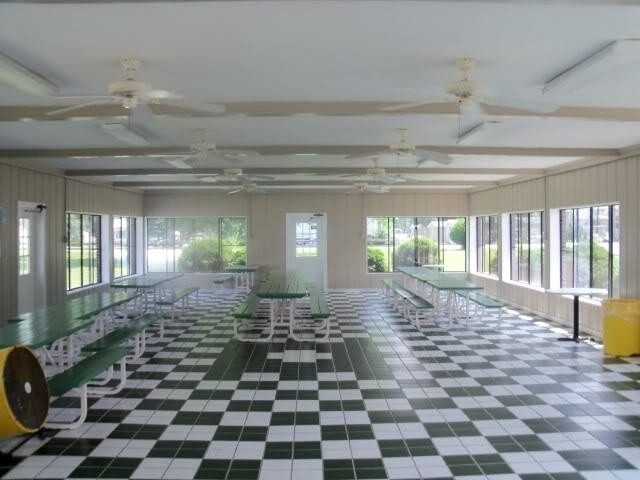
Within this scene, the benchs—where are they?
[154,286,202,324]
[304,281,332,346]
[381,278,436,339]
[230,285,261,346]
[213,272,234,296]
[45,347,131,431]
[78,310,167,365]
[451,288,509,333]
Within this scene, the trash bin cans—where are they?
[601,299,640,356]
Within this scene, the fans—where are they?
[31,54,227,134]
[142,127,258,163]
[195,165,274,198]
[0,343,50,466]
[335,127,455,192]
[377,52,561,144]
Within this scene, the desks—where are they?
[6,289,146,365]
[110,272,186,324]
[545,287,609,346]
[394,264,485,337]
[224,263,263,299]
[256,267,310,344]
[0,308,95,403]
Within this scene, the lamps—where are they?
[101,117,152,147]
[164,155,194,171]
[1,47,59,102]
[539,36,639,101]
[451,118,503,145]
